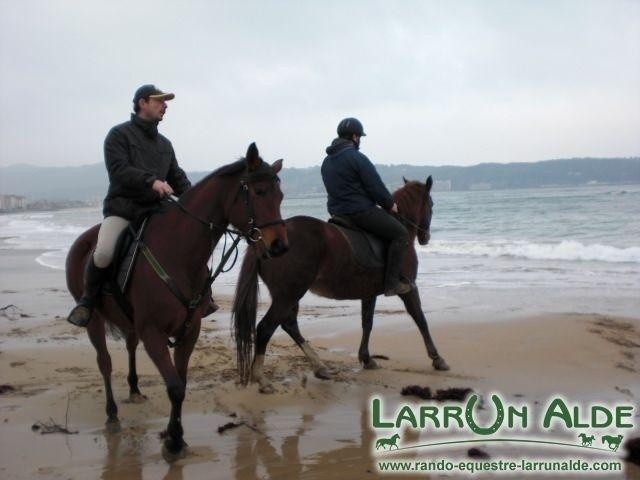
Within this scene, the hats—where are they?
[134,85,174,101]
[338,118,366,136]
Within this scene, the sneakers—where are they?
[385,282,410,296]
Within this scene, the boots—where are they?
[71,251,112,326]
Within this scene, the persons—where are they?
[67,84,218,327]
[321,117,416,297]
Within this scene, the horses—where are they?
[66,142,291,462]
[231,174,451,394]
